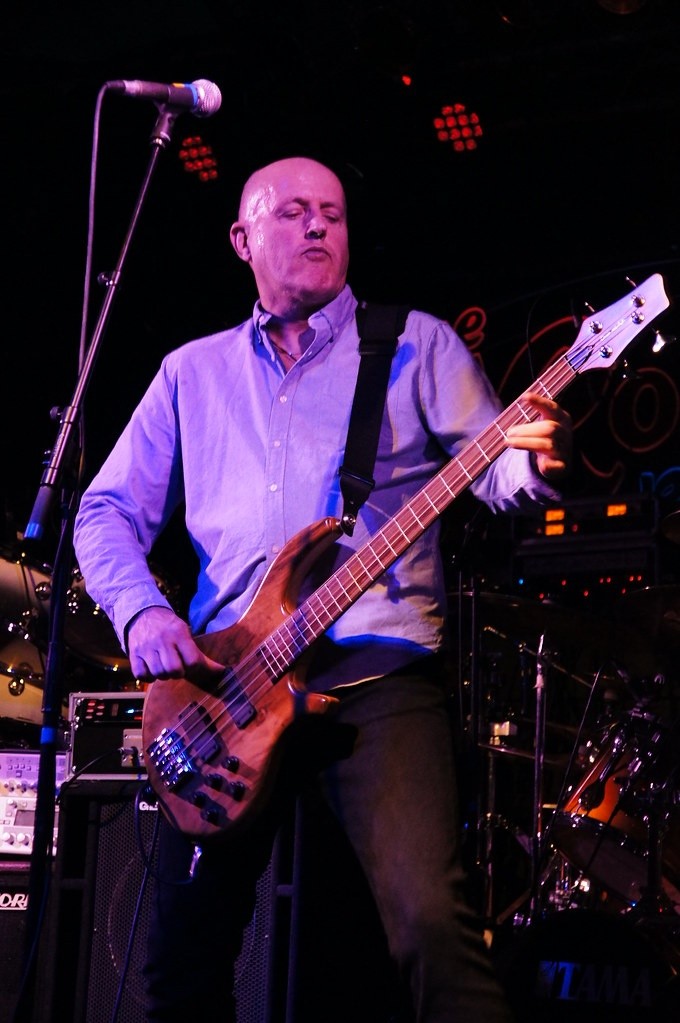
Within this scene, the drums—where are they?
[549,707,680,924]
[474,711,571,766]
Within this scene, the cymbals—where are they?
[615,582,680,639]
[659,507,680,544]
[441,587,590,641]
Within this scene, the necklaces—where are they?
[270,340,298,364]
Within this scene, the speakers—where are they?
[49,779,422,1023]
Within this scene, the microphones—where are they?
[105,78,223,118]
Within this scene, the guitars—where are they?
[143,265,676,848]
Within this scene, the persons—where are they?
[70,158,569,1023]
